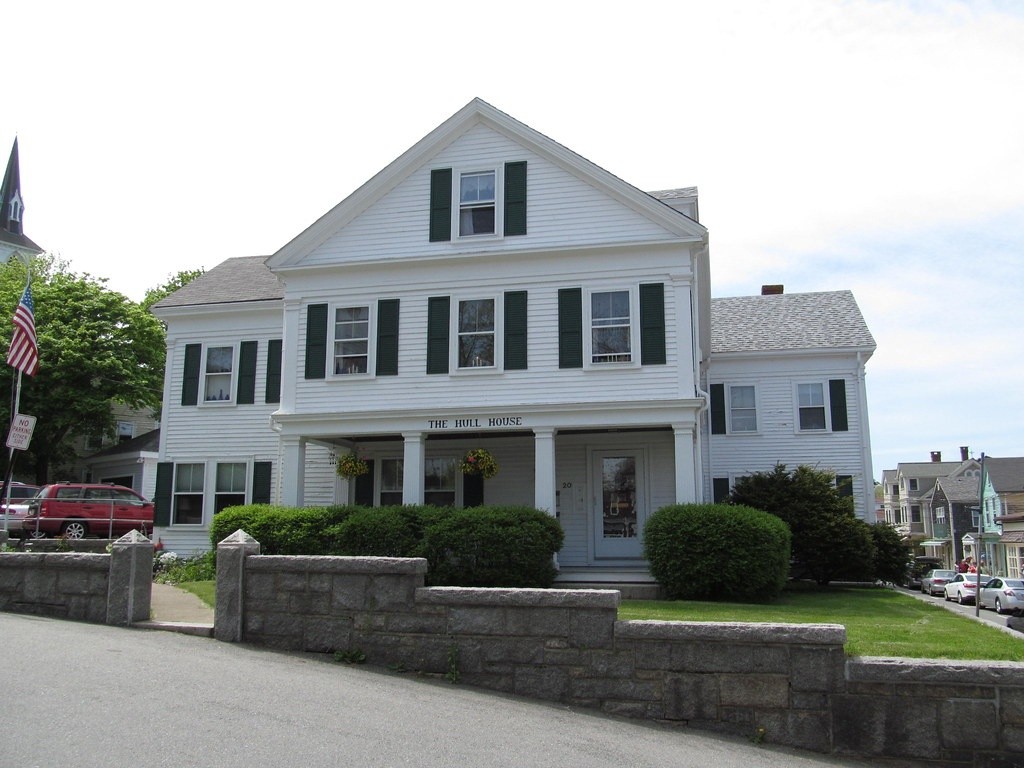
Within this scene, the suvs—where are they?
[22,480,156,540]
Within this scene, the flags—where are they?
[6,281,38,377]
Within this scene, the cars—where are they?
[0,481,42,539]
[943,572,994,605]
[975,576,1024,615]
[920,568,961,596]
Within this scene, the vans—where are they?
[902,555,946,590]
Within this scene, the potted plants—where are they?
[337,454,370,480]
[458,448,500,480]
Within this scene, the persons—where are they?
[955,557,1024,579]
